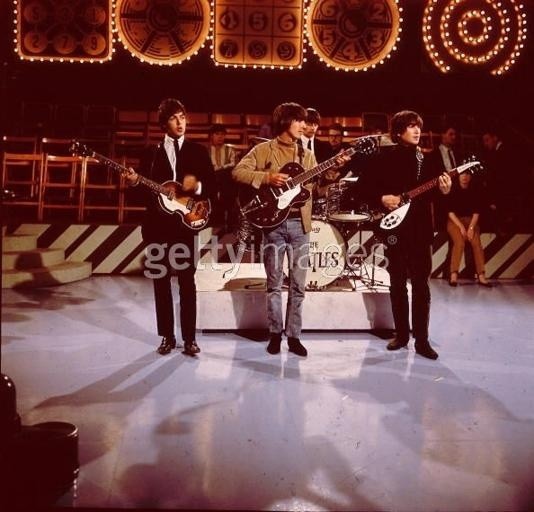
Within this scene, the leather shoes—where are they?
[159,337,200,354]
[268,335,307,356]
[387,339,437,360]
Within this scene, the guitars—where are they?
[369,156,483,247]
[239,137,377,230]
[67,135,211,231]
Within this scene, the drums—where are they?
[265,218,351,291]
[311,198,327,220]
[325,181,372,223]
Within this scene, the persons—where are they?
[295,107,341,204]
[353,110,451,360]
[120,98,217,355]
[328,123,343,153]
[426,123,461,175]
[230,100,352,357]
[441,169,493,289]
[479,129,518,231]
[202,121,236,173]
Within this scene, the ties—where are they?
[174,140,180,158]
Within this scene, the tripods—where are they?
[336,228,372,289]
[356,230,391,290]
